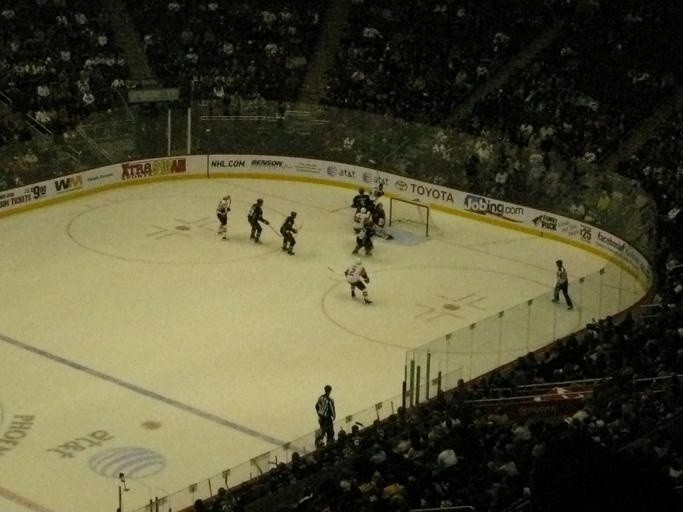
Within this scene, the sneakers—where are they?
[348,286,373,302]
[216,225,296,255]
[550,296,573,310]
[352,250,374,256]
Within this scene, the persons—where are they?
[0,0,682,256]
[193,258,680,511]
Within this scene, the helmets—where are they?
[358,189,363,193]
[354,258,360,265]
[556,260,563,265]
[290,210,297,216]
[324,385,332,391]
[360,207,366,212]
[256,199,263,203]
